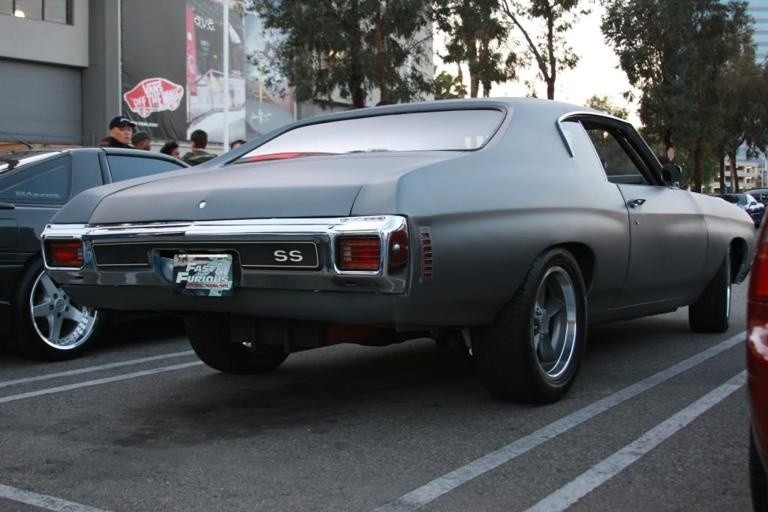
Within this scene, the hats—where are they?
[109,116,135,130]
[131,132,151,144]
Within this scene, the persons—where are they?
[95,116,248,167]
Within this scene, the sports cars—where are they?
[39,97,761,404]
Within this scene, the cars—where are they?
[1,146,191,356]
[710,193,765,227]
[745,218,767,512]
